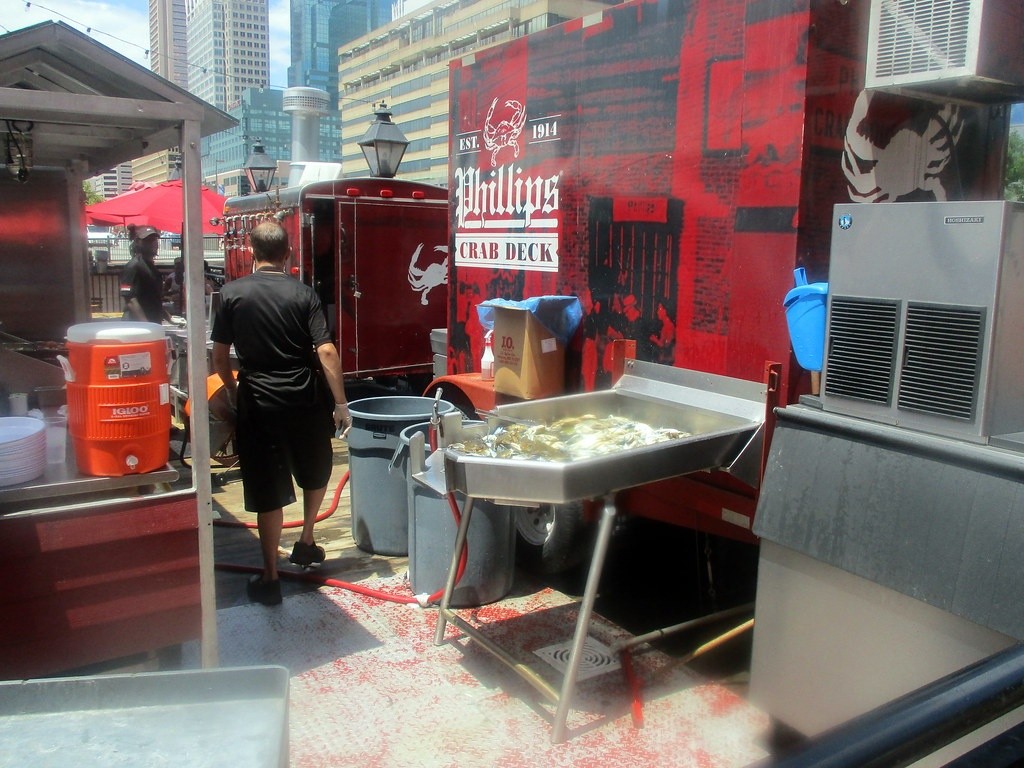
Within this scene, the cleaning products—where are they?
[480,330,494,381]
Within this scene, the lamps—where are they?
[6,120,34,182]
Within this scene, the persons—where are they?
[119,223,214,325]
[211,222,353,603]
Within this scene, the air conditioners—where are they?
[864,1,1023,103]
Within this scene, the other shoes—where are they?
[289,542,326,565]
[246,575,283,606]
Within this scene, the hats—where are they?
[134,226,161,240]
[174,257,182,264]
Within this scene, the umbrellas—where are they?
[83,178,228,317]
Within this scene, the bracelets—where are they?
[335,401,349,407]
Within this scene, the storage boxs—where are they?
[475,303,565,400]
[429,328,447,381]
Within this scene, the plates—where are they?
[0,417,47,486]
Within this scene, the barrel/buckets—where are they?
[58,322,172,476]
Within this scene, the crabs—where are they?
[447,414,691,463]
